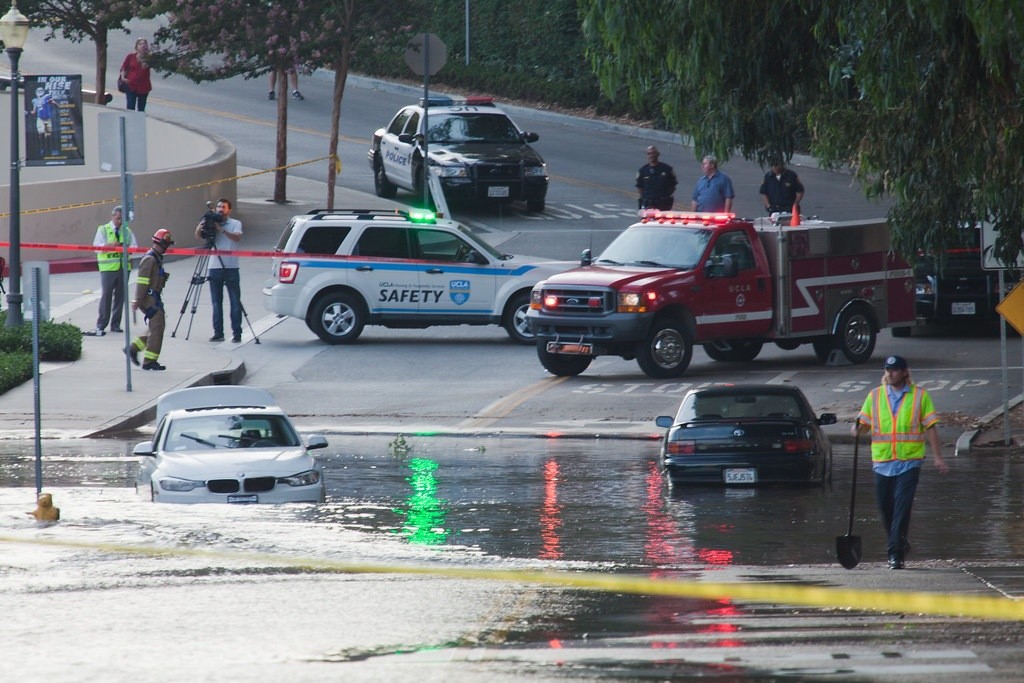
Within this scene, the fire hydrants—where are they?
[28,490,62,523]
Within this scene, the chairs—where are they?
[241,430,261,448]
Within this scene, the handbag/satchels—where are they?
[117,74,128,93]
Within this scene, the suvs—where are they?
[260,208,592,346]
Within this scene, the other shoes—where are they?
[123,347,140,366]
[210,335,224,342]
[232,337,241,343]
[111,326,123,332]
[142,361,165,370]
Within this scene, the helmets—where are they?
[151,228,174,245]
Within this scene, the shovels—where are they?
[835,418,862,569]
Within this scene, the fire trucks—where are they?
[524,208,917,382]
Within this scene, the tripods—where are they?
[172,230,260,344]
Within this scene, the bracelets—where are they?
[222,229,225,233]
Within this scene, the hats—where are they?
[881,356,906,368]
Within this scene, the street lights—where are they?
[0,1,33,333]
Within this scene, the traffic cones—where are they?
[789,205,801,227]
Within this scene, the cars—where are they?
[656,384,838,487]
[130,385,330,505]
[366,95,548,213]
[891,214,1024,339]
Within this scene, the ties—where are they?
[115,228,119,241]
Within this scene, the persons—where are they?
[93,205,138,336]
[850,356,948,569]
[123,229,174,371]
[31,86,57,159]
[691,155,736,213]
[120,38,152,112]
[266,67,304,100]
[634,146,678,211]
[194,198,244,343]
[759,158,805,217]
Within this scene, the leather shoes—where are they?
[887,556,904,570]
